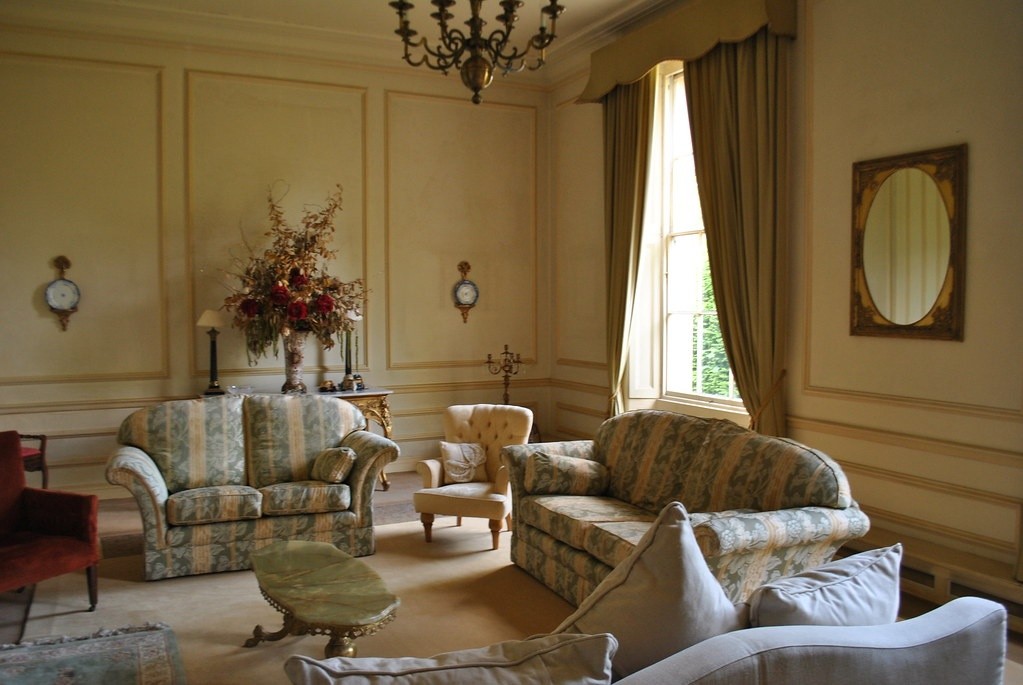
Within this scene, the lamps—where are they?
[388,0,567,104]
[197,309,227,395]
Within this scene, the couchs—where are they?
[611,594,1008,685]
[503,407,871,607]
[104,391,402,581]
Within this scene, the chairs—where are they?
[0,430,102,611]
[413,403,534,548]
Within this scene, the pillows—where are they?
[748,543,904,628]
[522,501,749,684]
[311,448,357,484]
[283,632,618,685]
[523,452,609,496]
[438,440,489,484]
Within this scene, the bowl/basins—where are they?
[226,386,256,394]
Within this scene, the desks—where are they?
[229,388,394,491]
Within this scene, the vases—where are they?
[278,323,308,394]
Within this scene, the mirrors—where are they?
[850,142,967,343]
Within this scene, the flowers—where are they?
[197,176,373,368]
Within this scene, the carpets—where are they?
[0,620,188,685]
[19,515,1022,685]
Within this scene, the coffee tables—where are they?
[243,539,401,657]
